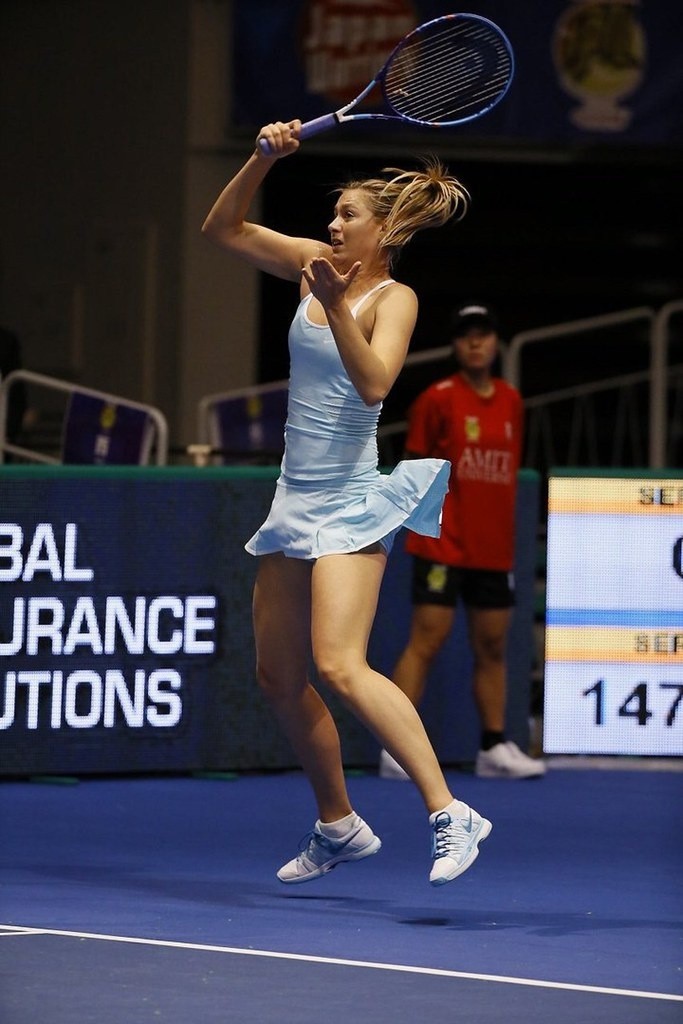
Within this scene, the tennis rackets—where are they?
[258,11,516,159]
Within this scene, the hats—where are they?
[451,302,496,338]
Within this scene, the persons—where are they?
[201,120,492,887]
[376,304,550,783]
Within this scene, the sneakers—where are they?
[429,801,493,887]
[475,740,546,780]
[379,748,412,781]
[277,819,382,884]
